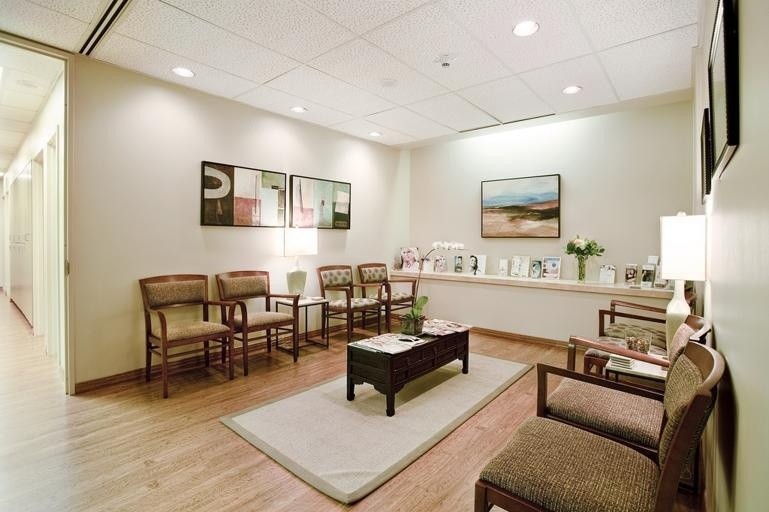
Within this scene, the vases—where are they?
[399,313,425,335]
[577,255,587,281]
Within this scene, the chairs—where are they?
[138,262,416,400]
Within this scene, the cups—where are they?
[623,328,653,355]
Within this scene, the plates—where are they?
[311,297,324,301]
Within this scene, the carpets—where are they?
[217,351,535,503]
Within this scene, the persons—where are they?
[399,247,560,281]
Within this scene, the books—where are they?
[422,318,472,338]
[354,332,426,356]
[610,353,636,370]
[598,264,616,284]
[623,255,667,290]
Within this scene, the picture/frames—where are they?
[540,256,561,280]
[699,0,739,203]
[480,173,561,239]
[200,160,352,230]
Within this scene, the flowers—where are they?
[401,240,464,319]
[564,234,606,280]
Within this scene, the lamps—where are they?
[659,211,706,361]
